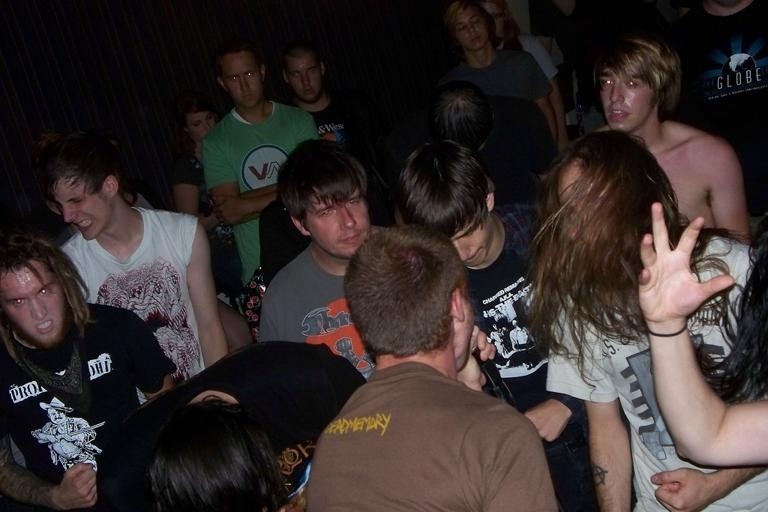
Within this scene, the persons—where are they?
[2,0,768,510]
